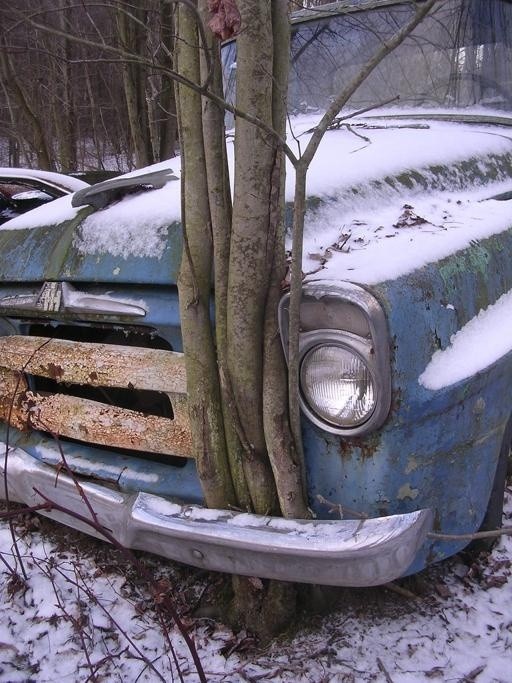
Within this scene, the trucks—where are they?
[1,0,510,591]
